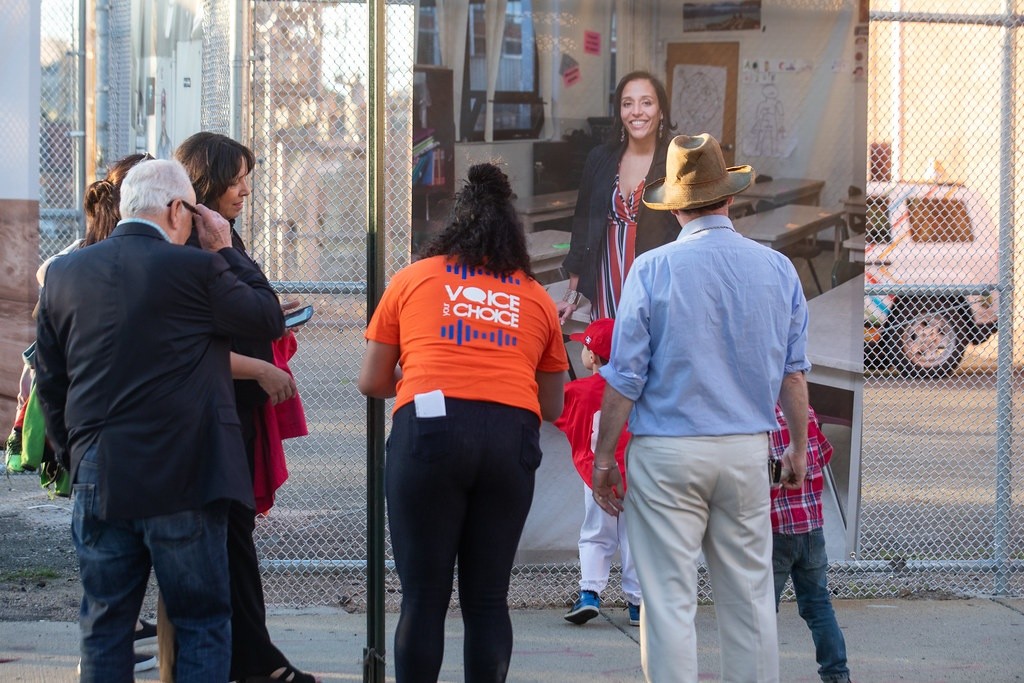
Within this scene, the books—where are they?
[412,126,446,187]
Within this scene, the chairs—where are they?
[847,186,865,234]
[831,259,864,288]
[755,199,824,294]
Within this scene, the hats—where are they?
[640,131,755,211]
[569,317,616,362]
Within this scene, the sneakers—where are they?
[622,602,640,626]
[563,590,600,625]
[133,617,158,648]
[133,652,157,673]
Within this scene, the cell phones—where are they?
[284,305,314,329]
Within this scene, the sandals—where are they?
[236,652,323,683]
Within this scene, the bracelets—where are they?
[563,288,582,306]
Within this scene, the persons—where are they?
[767,387,853,682]
[359,160,570,683]
[548,71,682,326]
[29,131,320,683]
[589,131,813,683]
[548,317,643,627]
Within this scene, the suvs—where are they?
[865,176,1002,378]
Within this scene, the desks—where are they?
[511,179,864,561]
